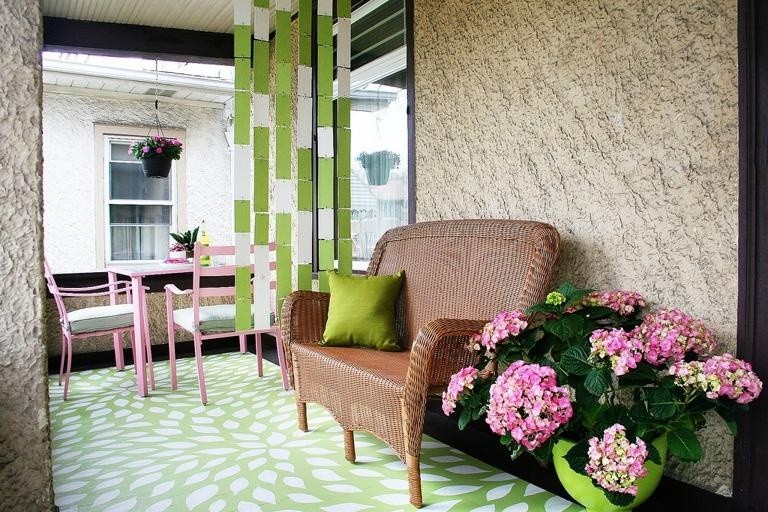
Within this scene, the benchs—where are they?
[279,219,561,508]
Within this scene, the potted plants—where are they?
[169,226,200,259]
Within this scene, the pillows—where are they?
[316,267,405,351]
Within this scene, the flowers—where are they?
[126,134,183,161]
[442,280,763,508]
[355,149,401,169]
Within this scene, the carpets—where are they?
[47,351,591,512]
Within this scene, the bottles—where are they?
[196,222,211,266]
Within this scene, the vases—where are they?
[141,156,171,179]
[552,432,668,512]
[365,164,389,185]
